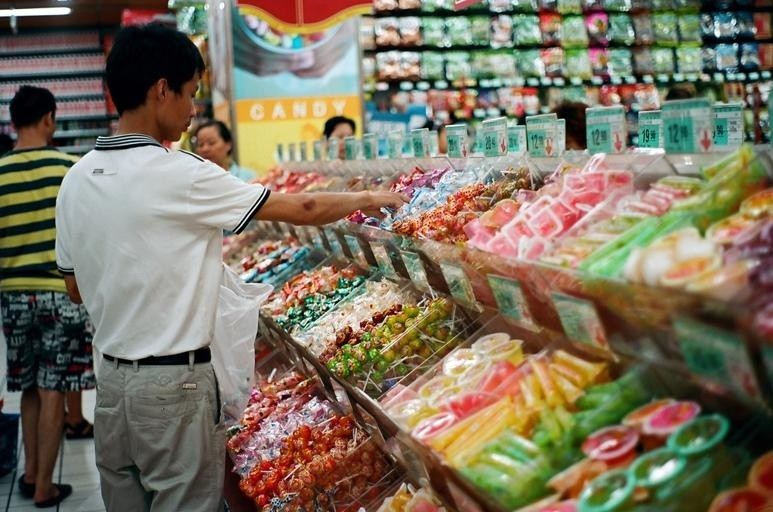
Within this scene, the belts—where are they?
[102,345,212,365]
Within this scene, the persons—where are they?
[55,24,405,512]
[65,391,93,440]
[549,102,596,154]
[192,121,257,238]
[0,84,84,508]
[0,130,22,478]
[319,115,359,159]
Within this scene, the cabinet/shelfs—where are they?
[0,1,108,154]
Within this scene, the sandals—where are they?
[65,419,94,440]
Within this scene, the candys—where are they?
[222,164,561,512]
[242,14,324,50]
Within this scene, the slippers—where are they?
[35,484,72,508]
[18,473,36,498]
